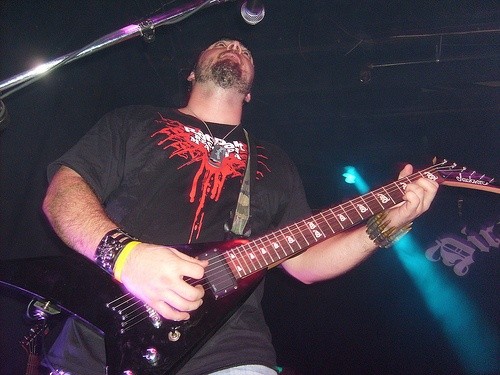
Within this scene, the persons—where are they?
[42,39,439,375]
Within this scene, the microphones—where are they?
[0,99,10,131]
[241,0,265,25]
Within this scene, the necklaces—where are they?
[188,105,241,168]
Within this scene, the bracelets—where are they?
[364,211,413,248]
[92,229,142,283]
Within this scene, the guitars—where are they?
[19,321,49,375]
[95,156,500,375]
